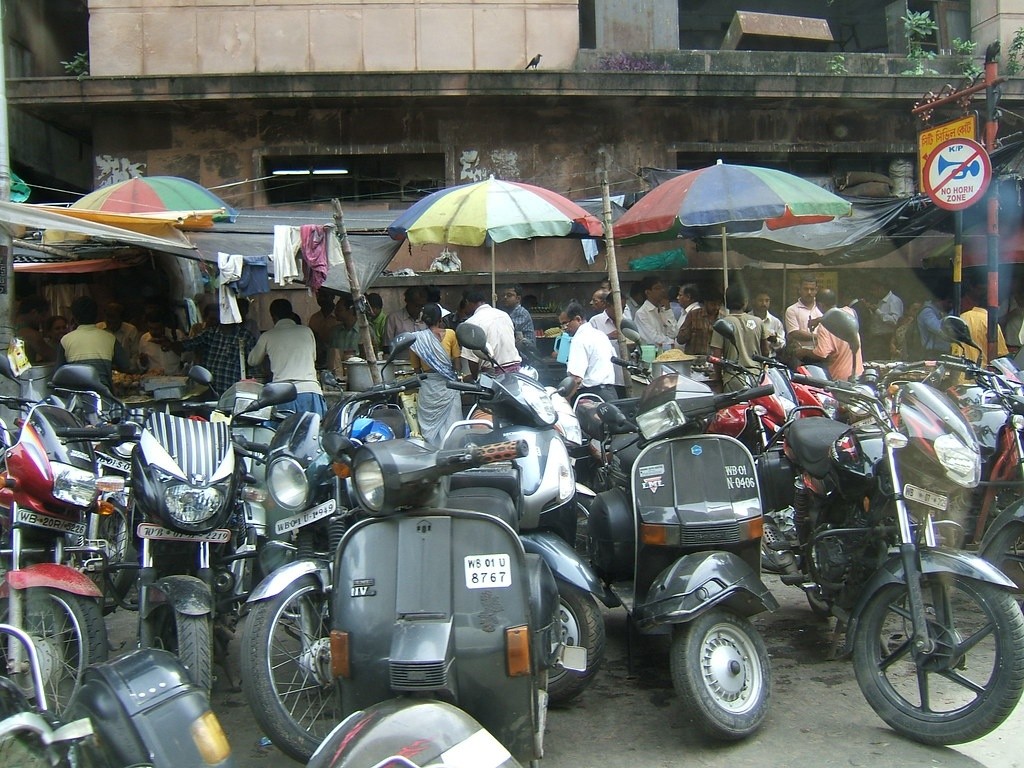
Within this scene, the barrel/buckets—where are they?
[651,358,693,379]
[641,344,656,370]
[554,333,572,364]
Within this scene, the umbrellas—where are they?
[41,175,233,341]
[613,160,853,314]
[386,174,606,310]
[920,213,1024,271]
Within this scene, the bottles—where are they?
[657,342,663,357]
[344,349,356,359]
[358,342,366,359]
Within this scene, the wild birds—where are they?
[525,54,543,70]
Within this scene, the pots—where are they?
[791,341,824,362]
[21,359,57,401]
[343,360,396,391]
[393,360,414,371]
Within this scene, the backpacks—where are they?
[905,306,939,361]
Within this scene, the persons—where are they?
[0,274,1024,446]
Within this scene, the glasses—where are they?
[560,318,575,329]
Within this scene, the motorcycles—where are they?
[1,306,1023,768]
